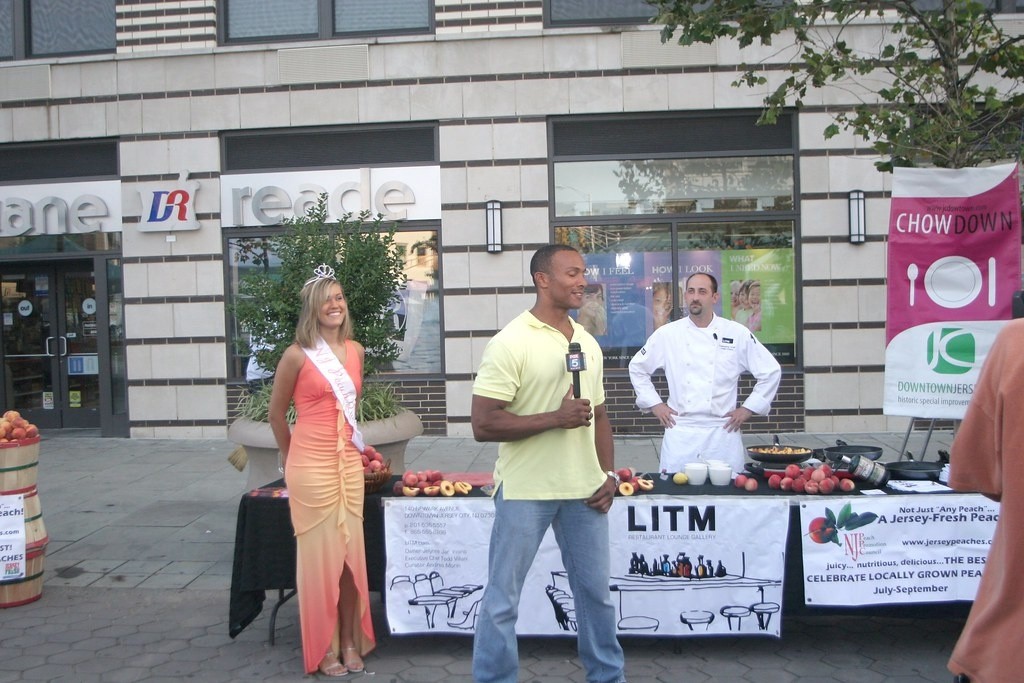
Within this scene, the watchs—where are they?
[607,470,620,488]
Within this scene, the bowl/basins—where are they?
[684,460,731,485]
[480,485,495,495]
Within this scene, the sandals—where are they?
[318,651,348,676]
[341,647,364,672]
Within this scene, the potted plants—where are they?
[229,192,424,492]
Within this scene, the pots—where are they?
[822,439,883,463]
[746,435,812,463]
[885,451,944,479]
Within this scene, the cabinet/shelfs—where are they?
[4,340,44,409]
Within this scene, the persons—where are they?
[576,284,605,335]
[653,281,674,332]
[729,279,761,331]
[470,244,626,683]
[947,317,1024,683]
[268,262,376,677]
[628,272,781,480]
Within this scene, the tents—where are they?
[0,235,122,283]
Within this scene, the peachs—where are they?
[809,517,834,543]
[361,445,472,496]
[611,468,654,496]
[0,411,39,442]
[735,465,855,493]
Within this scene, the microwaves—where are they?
[743,461,885,479]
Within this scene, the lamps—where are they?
[848,189,866,245]
[485,200,503,253]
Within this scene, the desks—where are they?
[229,473,1003,647]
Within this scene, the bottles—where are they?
[630,552,726,577]
[836,454,890,487]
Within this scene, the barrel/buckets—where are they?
[22,489,48,550]
[0,436,40,495]
[0,542,48,608]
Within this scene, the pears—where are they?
[673,473,690,484]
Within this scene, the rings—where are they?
[588,412,591,420]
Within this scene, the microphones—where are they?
[565,342,587,398]
